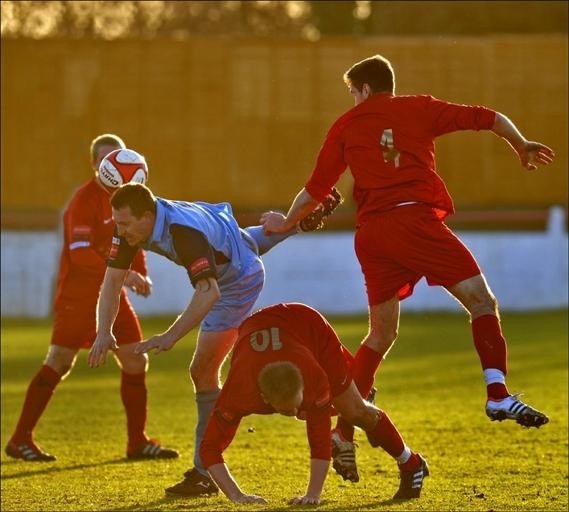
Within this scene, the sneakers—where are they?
[393,453,429,499]
[296,187,343,234]
[5,441,56,462]
[486,392,548,427]
[165,468,218,496]
[329,429,360,482]
[127,439,177,458]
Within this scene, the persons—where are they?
[195,300,434,508]
[257,51,556,484]
[85,182,347,500]
[5,132,181,465]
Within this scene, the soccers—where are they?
[98,148,149,193]
